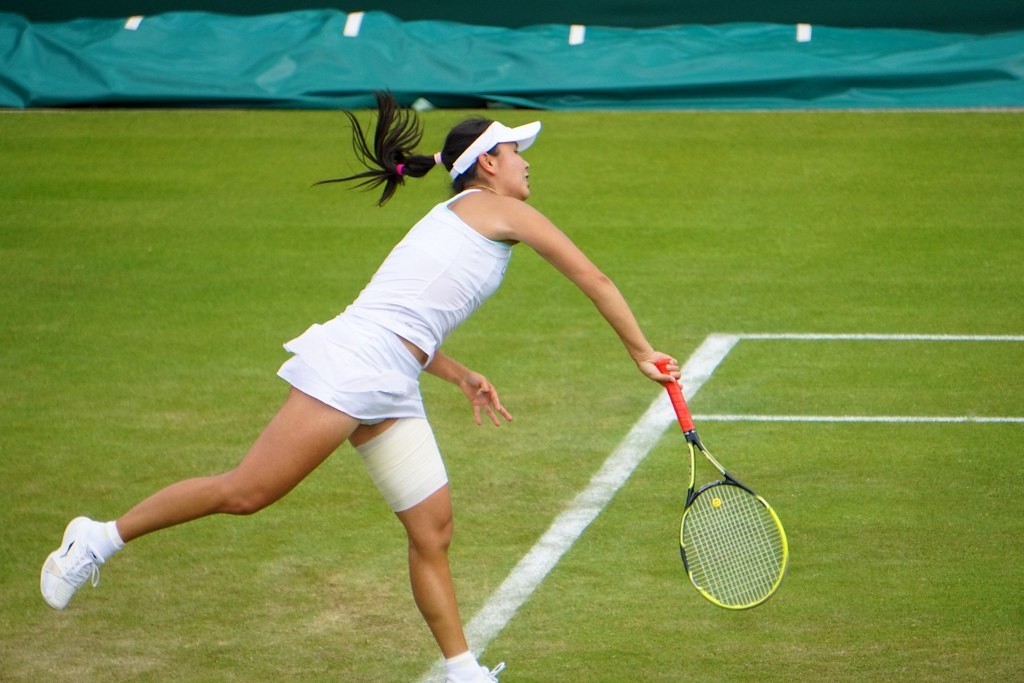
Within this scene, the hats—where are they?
[449,120,542,181]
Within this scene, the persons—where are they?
[39,87,681,683]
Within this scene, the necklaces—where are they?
[465,185,498,195]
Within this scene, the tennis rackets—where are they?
[655,358,790,611]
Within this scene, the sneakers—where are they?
[444,661,506,683]
[39,516,107,611]
